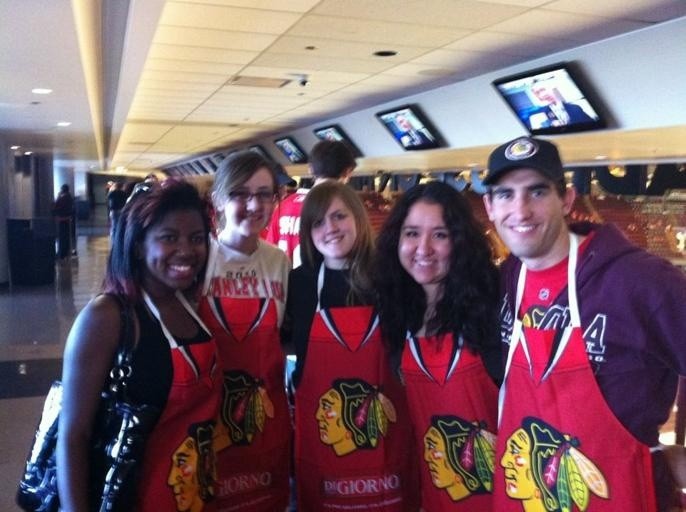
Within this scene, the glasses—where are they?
[225,190,273,204]
[126,182,151,205]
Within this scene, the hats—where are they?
[481,137,563,185]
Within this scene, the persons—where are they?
[324,131,337,142]
[393,113,431,148]
[423,413,498,501]
[266,141,358,260]
[56,177,224,512]
[499,415,610,512]
[373,182,502,512]
[198,148,292,511]
[53,183,74,259]
[280,141,302,162]
[169,419,219,509]
[215,368,274,452]
[106,173,160,245]
[314,376,398,457]
[278,179,419,511]
[254,161,297,240]
[520,78,594,135]
[480,137,686,512]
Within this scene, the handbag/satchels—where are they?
[16,379,149,512]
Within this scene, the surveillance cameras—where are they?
[298,78,308,87]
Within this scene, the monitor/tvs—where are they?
[491,61,605,136]
[273,136,307,164]
[162,145,272,177]
[313,124,361,158]
[374,104,442,151]
